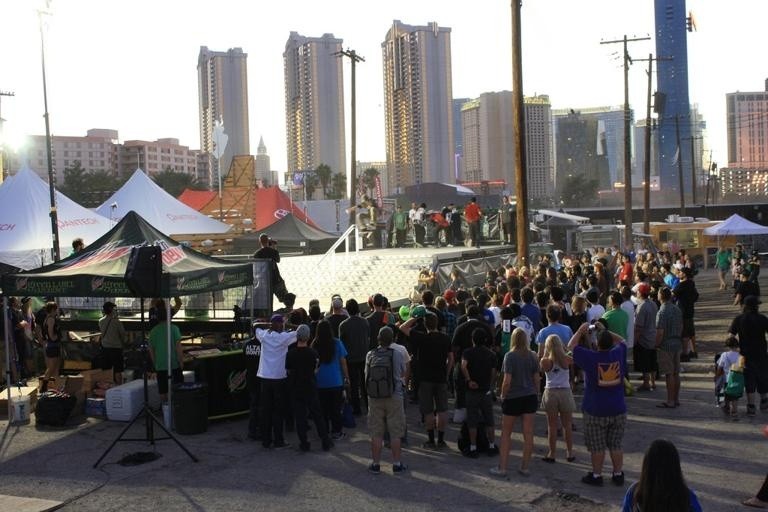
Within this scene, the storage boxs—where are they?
[0,386,38,414]
[38,374,65,391]
[86,398,105,417]
[80,367,115,397]
[106,378,161,421]
[36,396,76,426]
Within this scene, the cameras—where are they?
[268,239,277,246]
[589,324,597,330]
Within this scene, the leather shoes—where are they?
[567,456,576,463]
[741,495,768,508]
[541,456,556,464]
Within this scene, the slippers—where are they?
[655,402,681,409]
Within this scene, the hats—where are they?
[331,296,343,309]
[270,314,285,324]
[296,324,310,341]
[408,288,422,303]
[742,294,763,305]
[444,289,454,300]
[399,305,411,321]
[410,305,426,319]
[638,284,651,296]
[379,326,394,340]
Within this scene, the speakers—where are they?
[123,246,161,297]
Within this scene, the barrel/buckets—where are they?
[10,396,30,423]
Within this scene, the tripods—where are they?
[93,299,199,468]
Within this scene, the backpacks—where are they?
[723,354,746,400]
[364,347,396,400]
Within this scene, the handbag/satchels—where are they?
[339,385,357,429]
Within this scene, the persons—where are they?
[98,302,126,386]
[497,194,514,244]
[346,194,382,232]
[255,244,701,476]
[253,234,289,303]
[385,197,485,248]
[148,309,184,402]
[149,295,182,331]
[566,320,630,488]
[0,292,63,387]
[713,242,768,419]
[70,238,84,255]
[742,473,768,508]
[622,439,704,511]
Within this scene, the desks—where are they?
[182,344,259,422]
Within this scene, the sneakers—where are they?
[636,350,698,392]
[611,471,625,486]
[393,463,408,473]
[489,465,507,477]
[368,463,380,474]
[518,465,530,476]
[246,426,348,451]
[719,283,728,290]
[582,472,604,488]
[488,445,499,456]
[462,447,479,459]
[721,399,768,417]
[384,419,449,450]
